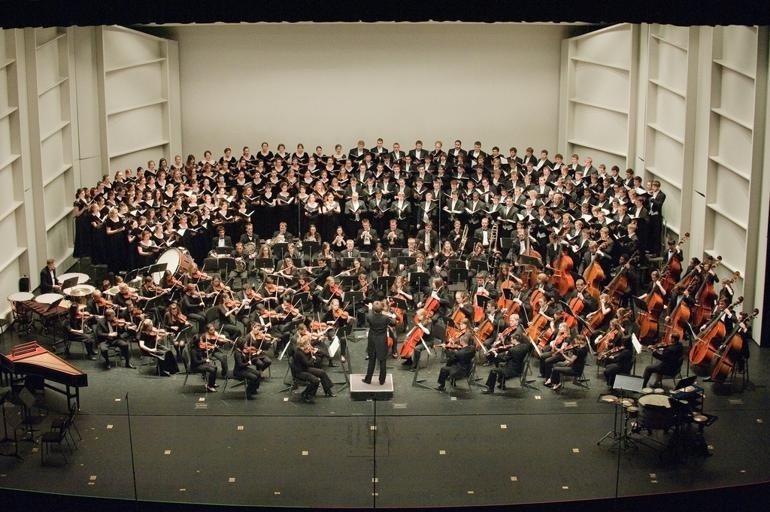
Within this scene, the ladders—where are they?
[75,271,372,356]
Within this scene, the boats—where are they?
[19,278,29,292]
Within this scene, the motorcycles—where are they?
[608,411,639,453]
[597,404,621,445]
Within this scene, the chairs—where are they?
[50,402,83,448]
[40,418,72,466]
[11,250,750,406]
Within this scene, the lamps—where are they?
[40,418,72,466]
[50,402,83,448]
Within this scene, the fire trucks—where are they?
[296,265,322,270]
[333,267,358,278]
[277,264,293,274]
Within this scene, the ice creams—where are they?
[336,233,343,247]
[364,227,370,245]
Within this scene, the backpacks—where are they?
[34,293,63,304]
[64,284,94,297]
[637,394,672,429]
[7,292,34,313]
[151,246,198,286]
[57,272,90,284]
[641,387,665,396]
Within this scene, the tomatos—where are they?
[0,340,88,413]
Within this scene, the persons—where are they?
[39,138,753,403]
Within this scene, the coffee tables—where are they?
[616,398,633,407]
[625,405,638,412]
[601,394,617,402]
